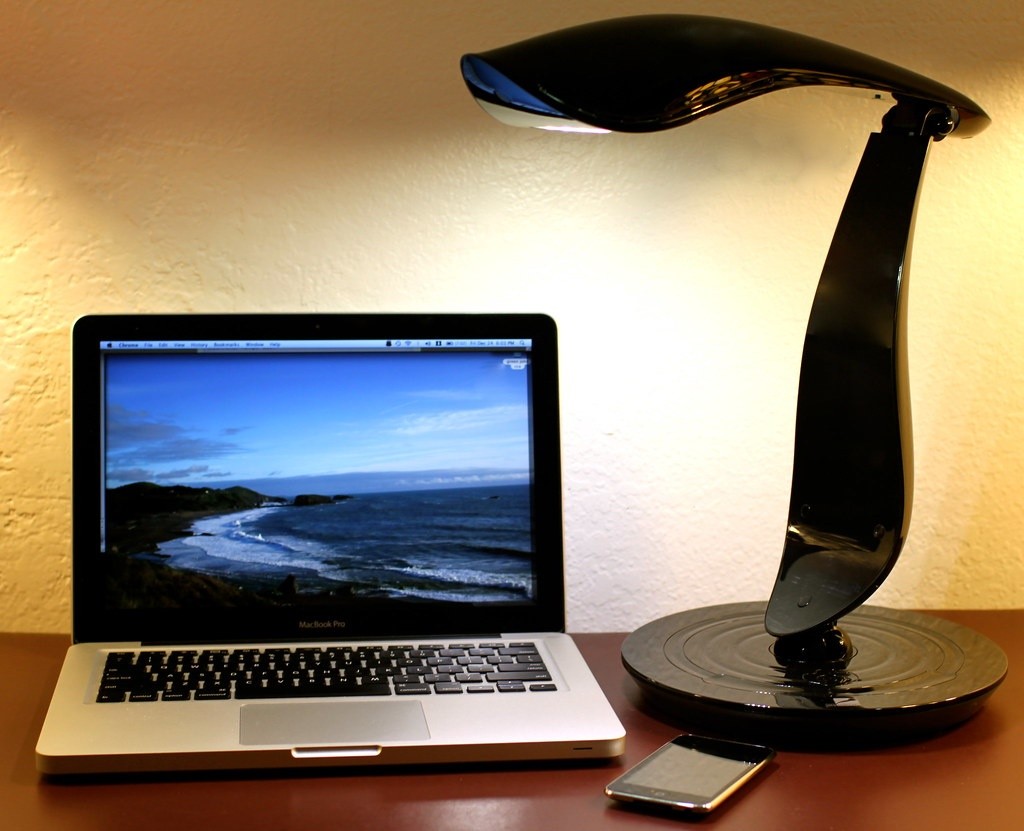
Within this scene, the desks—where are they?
[0,607,1024,831]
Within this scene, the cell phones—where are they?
[602,733,778,815]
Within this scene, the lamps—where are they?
[459,13,1007,745]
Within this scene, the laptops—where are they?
[34,312,626,774]
